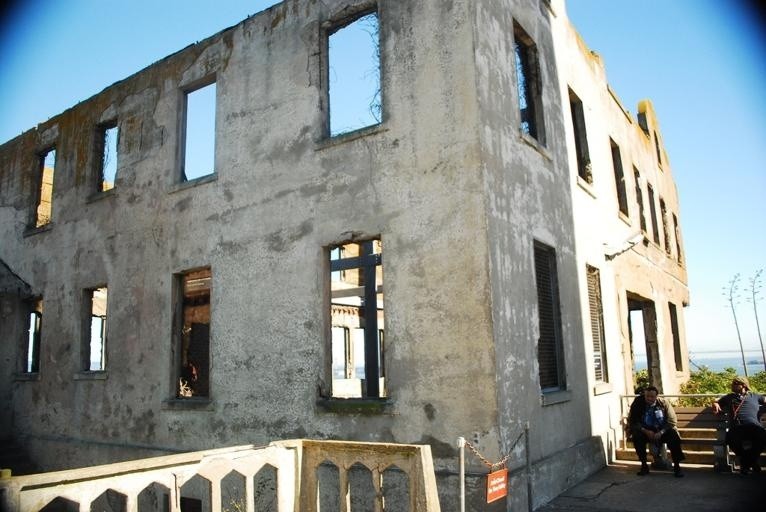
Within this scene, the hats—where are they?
[733,376,751,391]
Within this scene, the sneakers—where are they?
[636,467,650,476]
[739,456,762,475]
[674,468,684,478]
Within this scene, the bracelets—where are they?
[660,430,665,435]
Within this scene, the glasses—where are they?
[733,381,743,385]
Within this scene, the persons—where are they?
[709,375,766,475]
[628,386,689,476]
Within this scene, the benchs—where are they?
[616,404,765,473]
